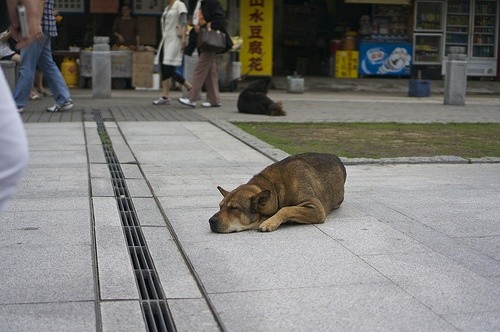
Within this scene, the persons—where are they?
[112,5,140,47]
[152,0,195,105]
[0,0,75,219]
[178,0,239,108]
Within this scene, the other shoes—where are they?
[152,96,172,106]
[200,101,222,107]
[178,97,197,108]
[28,89,53,100]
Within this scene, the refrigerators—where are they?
[358,38,412,78]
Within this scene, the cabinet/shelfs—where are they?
[371,4,409,35]
[279,4,320,50]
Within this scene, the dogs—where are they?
[236,76,287,116]
[207,152,347,234]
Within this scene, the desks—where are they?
[53,50,132,79]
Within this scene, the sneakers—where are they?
[46,99,74,112]
[16,106,24,113]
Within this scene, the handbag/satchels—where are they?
[195,20,228,54]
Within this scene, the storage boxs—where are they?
[335,49,358,78]
[408,78,432,97]
[130,51,156,88]
[286,76,306,93]
